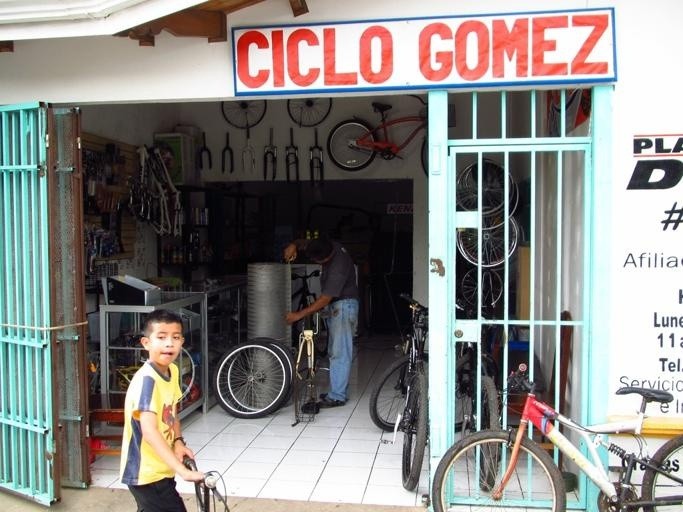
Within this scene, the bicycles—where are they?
[326,95,456,178]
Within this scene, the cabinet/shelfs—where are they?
[94,273,248,430]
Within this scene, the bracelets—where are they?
[173,436,186,446]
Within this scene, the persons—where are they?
[118,308,206,510]
[282,235,360,409]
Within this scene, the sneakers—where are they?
[317,399,348,409]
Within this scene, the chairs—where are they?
[486,310,574,477]
